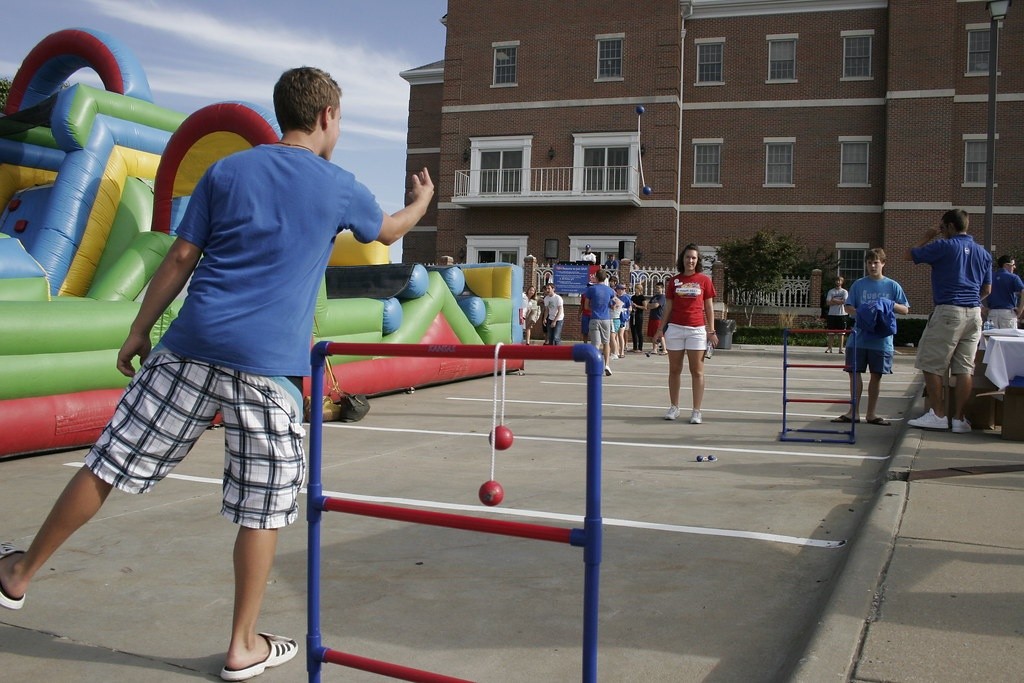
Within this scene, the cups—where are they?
[704,341,714,359]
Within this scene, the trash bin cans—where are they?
[713,319,734,349]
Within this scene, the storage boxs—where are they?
[975,383,1024,442]
[922,375,995,430]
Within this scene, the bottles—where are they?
[984,320,990,330]
[989,319,996,329]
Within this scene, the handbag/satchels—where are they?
[305,395,341,421]
[341,395,370,420]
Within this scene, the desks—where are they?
[978,328,1024,354]
[982,335,1024,391]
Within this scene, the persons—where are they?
[584,269,615,376]
[904,209,992,434]
[647,281,668,355]
[831,248,910,425]
[627,285,647,353]
[980,254,1024,329]
[604,254,617,270]
[520,277,632,359]
[578,244,596,265]
[652,243,718,423]
[825,277,848,354]
[0,65,434,682]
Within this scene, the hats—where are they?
[586,244,590,248]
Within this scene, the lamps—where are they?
[641,144,646,157]
[548,147,556,160]
[463,149,470,162]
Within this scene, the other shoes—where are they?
[605,366,612,376]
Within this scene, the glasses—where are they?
[1006,263,1015,266]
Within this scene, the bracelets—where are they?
[708,330,716,335]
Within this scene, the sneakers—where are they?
[690,411,701,423]
[951,417,971,433]
[907,408,948,429]
[665,405,680,419]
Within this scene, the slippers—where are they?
[830,415,860,423]
[0,543,25,609]
[867,418,891,426]
[220,632,298,681]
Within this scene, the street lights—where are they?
[983,0,1011,254]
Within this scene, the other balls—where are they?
[478,481,504,506]
[635,104,645,114]
[708,455,715,460]
[488,425,514,450]
[697,456,703,462]
[642,186,652,195]
